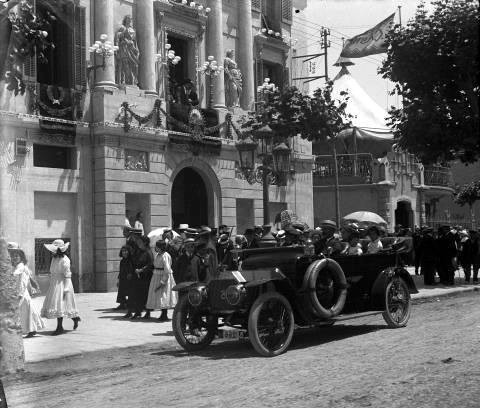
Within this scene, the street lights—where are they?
[235,124,294,248]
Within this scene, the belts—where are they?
[154,267,164,270]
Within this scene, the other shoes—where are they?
[51,317,64,336]
[71,316,81,330]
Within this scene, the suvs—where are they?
[169,231,419,358]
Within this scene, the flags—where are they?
[341,10,396,58]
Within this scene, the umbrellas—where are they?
[147,227,180,240]
[343,211,388,228]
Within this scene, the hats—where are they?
[121,218,132,228]
[43,239,70,254]
[184,228,199,235]
[183,238,199,246]
[178,223,189,230]
[243,228,255,235]
[276,229,286,239]
[199,227,211,235]
[319,219,337,229]
[129,227,143,234]
[218,233,229,245]
[6,241,23,250]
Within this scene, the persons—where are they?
[6,242,22,250]
[10,249,45,337]
[0,237,25,408]
[116,224,263,322]
[142,240,178,321]
[273,220,412,266]
[224,49,242,107]
[178,78,199,106]
[134,211,145,236]
[125,210,132,228]
[116,14,140,86]
[413,225,480,286]
[173,237,203,337]
[40,239,81,336]
